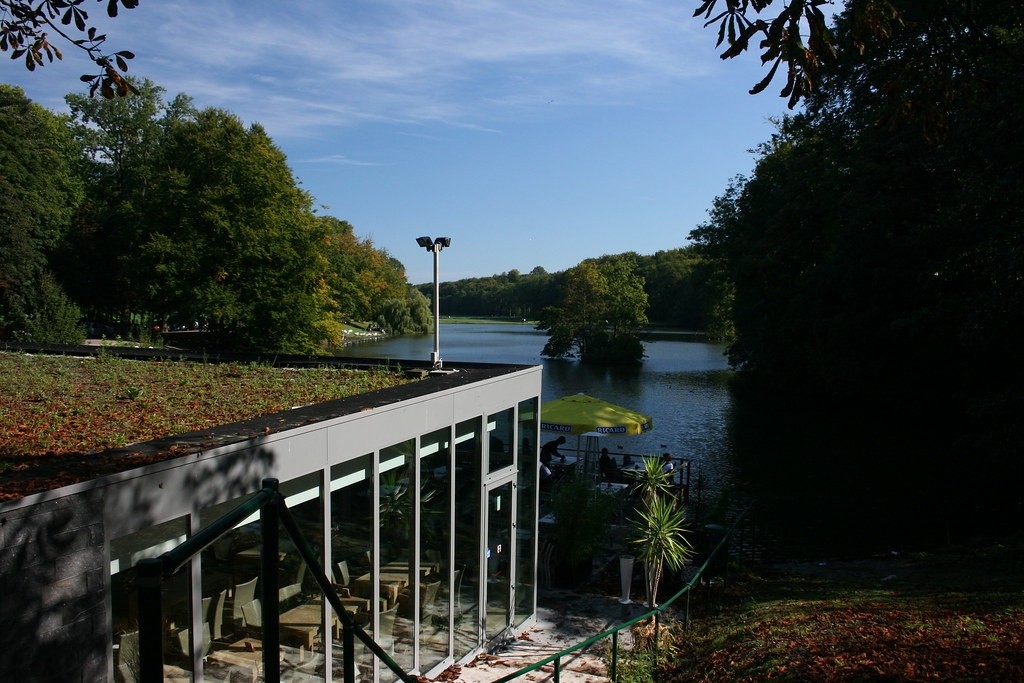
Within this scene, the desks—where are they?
[549,456,585,470]
[279,593,370,651]
[354,561,439,606]
[210,637,299,680]
[593,482,628,496]
[237,543,288,561]
[621,462,649,485]
[539,512,559,526]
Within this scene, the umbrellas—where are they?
[518,393,653,479]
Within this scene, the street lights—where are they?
[416,235,452,368]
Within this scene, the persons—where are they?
[151,323,160,343]
[366,320,386,334]
[540,436,566,470]
[661,453,673,485]
[599,448,636,487]
[172,317,218,331]
[522,437,533,456]
[162,323,169,334]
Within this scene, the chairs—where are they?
[116,550,467,683]
